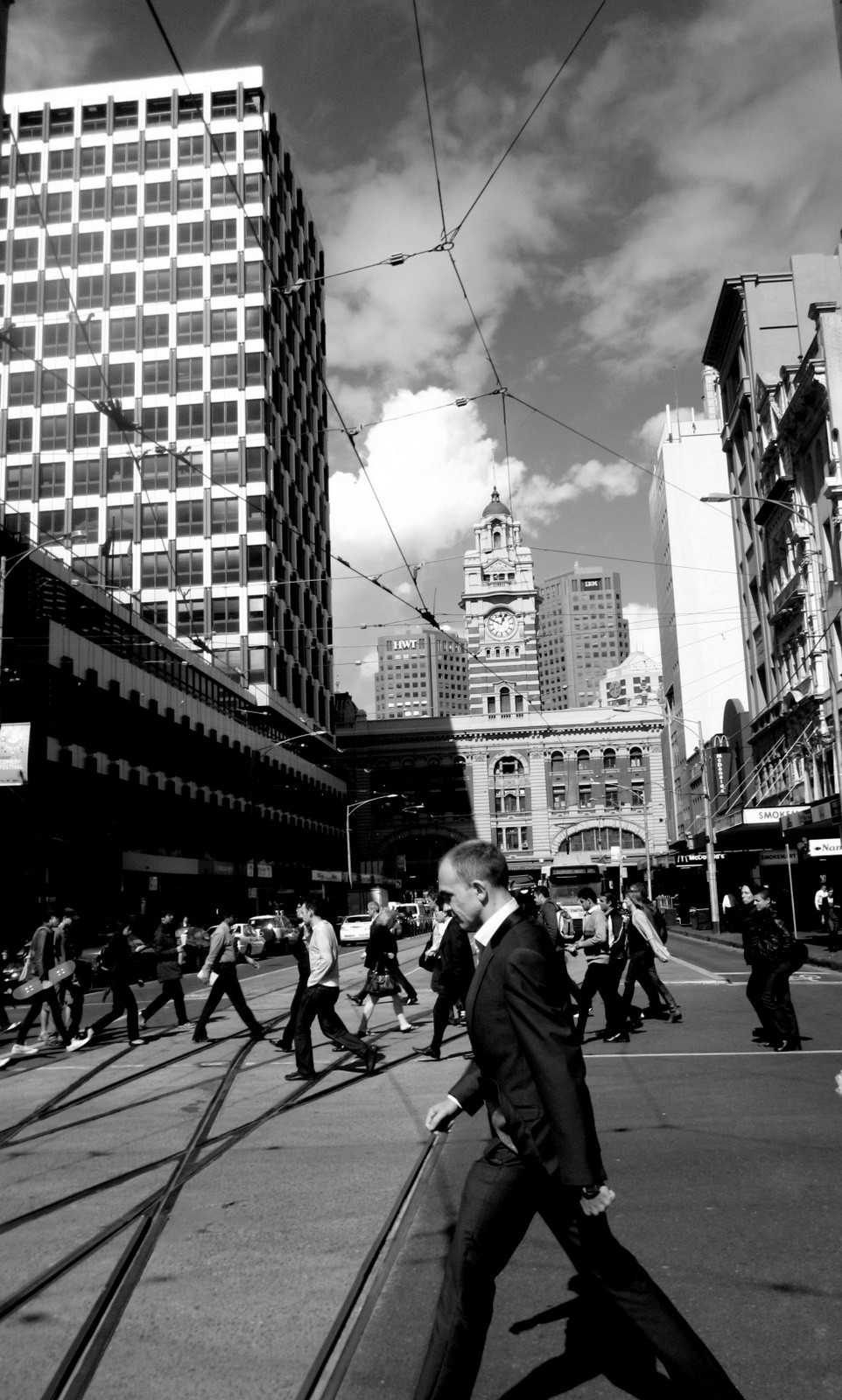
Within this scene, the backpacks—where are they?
[540,899,574,939]
[651,909,667,943]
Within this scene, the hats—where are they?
[62,907,80,920]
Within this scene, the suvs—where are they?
[246,914,301,955]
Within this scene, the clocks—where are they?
[484,609,517,641]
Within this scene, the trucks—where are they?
[508,854,614,941]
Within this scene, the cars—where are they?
[394,902,436,936]
[339,914,404,947]
[0,923,268,1001]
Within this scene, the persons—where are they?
[412,841,748,1399]
[813,884,840,952]
[722,882,808,1052]
[0,892,475,1080]
[532,882,683,1042]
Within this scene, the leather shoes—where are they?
[412,1046,440,1059]
[270,1039,291,1052]
[604,1030,630,1043]
[332,1044,350,1051]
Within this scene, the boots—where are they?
[661,987,682,1023]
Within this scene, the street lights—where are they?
[250,730,328,915]
[394,804,426,880]
[611,704,719,934]
[588,781,652,903]
[346,792,400,891]
[699,489,842,797]
[589,796,623,903]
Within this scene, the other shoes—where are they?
[253,1026,270,1040]
[138,1011,147,1028]
[358,1030,375,1036]
[404,997,417,1005]
[129,1039,149,1046]
[178,1022,197,1028]
[400,1026,419,1032]
[193,1035,210,1042]
[630,1006,642,1029]
[762,1035,804,1052]
[365,1045,378,1075]
[347,994,363,1006]
[4,1021,21,1033]
[38,1032,58,1042]
[285,1071,316,1080]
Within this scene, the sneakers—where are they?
[11,1044,38,1057]
[66,1037,91,1052]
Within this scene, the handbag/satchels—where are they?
[19,949,40,981]
[419,941,442,972]
[365,952,401,997]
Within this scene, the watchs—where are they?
[581,1182,605,1199]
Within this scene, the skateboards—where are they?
[12,960,76,1000]
